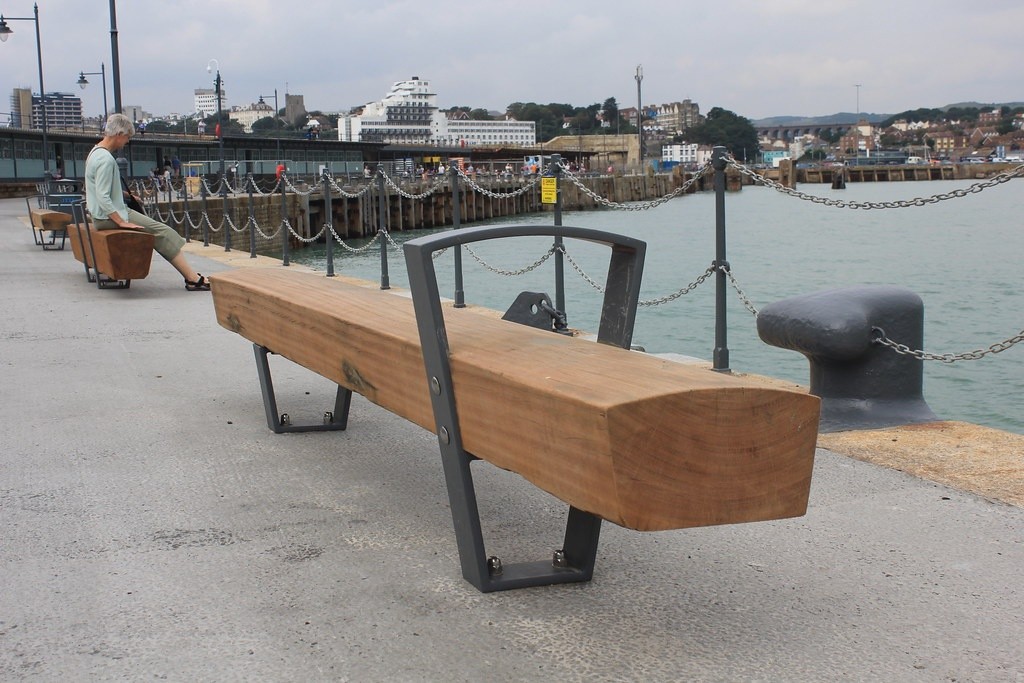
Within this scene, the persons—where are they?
[198,118,208,138]
[148,167,171,188]
[99,115,107,131]
[417,163,513,180]
[305,125,321,140]
[275,161,285,190]
[137,120,146,138]
[164,155,182,178]
[363,166,371,178]
[607,165,612,176]
[565,161,585,174]
[520,163,536,178]
[85,114,211,291]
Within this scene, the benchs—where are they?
[207,269,823,592]
[30,208,73,251]
[66,222,156,290]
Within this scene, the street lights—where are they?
[76,62,108,121]
[0,2,50,183]
[635,66,645,174]
[258,89,281,160]
[207,59,227,194]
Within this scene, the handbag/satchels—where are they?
[125,195,145,214]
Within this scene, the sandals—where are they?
[185,273,210,290]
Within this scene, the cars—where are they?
[907,153,1023,163]
